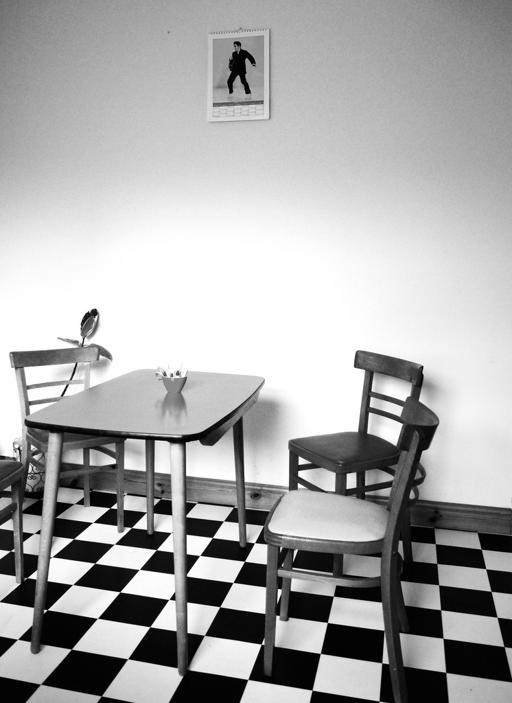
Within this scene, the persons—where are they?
[227,41,257,95]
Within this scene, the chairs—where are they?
[263,397,440,703]
[288,350,423,571]
[10,344,127,533]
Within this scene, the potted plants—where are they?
[14,307,113,498]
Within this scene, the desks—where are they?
[23,368,269,679]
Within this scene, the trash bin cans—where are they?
[13,438,47,493]
